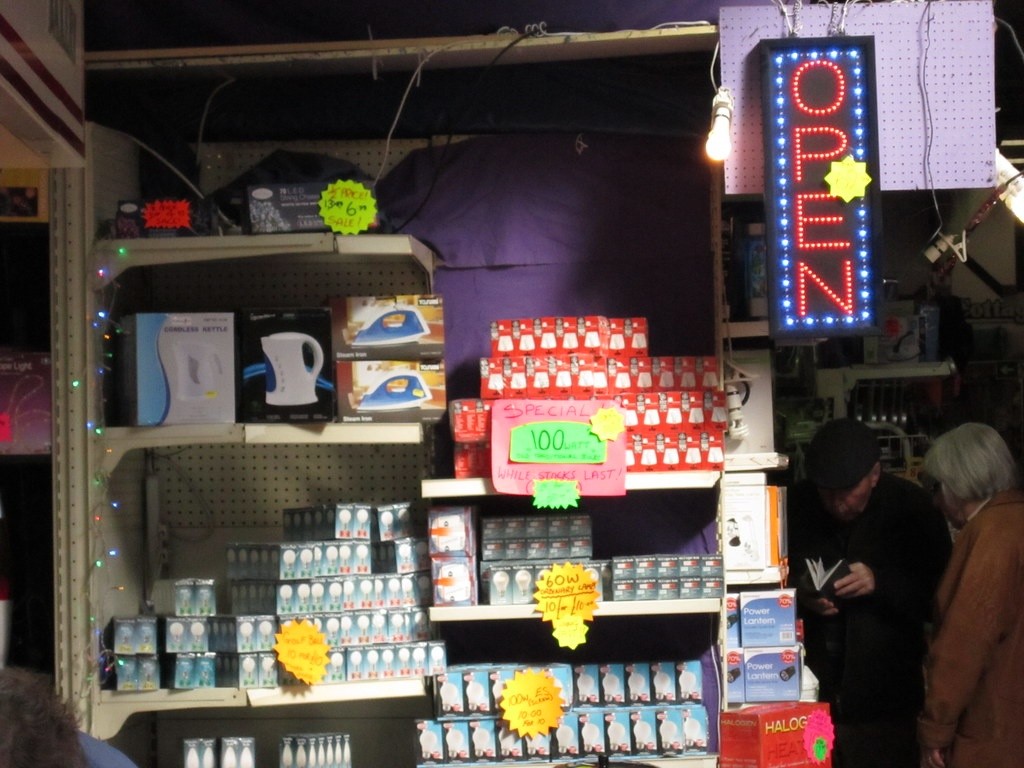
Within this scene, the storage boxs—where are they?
[112,179,833,767]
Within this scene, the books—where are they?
[804,556,859,609]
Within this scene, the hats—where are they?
[805,417,882,490]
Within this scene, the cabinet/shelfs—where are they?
[46,26,725,768]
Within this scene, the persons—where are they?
[917,423,1024,768]
[787,417,954,767]
